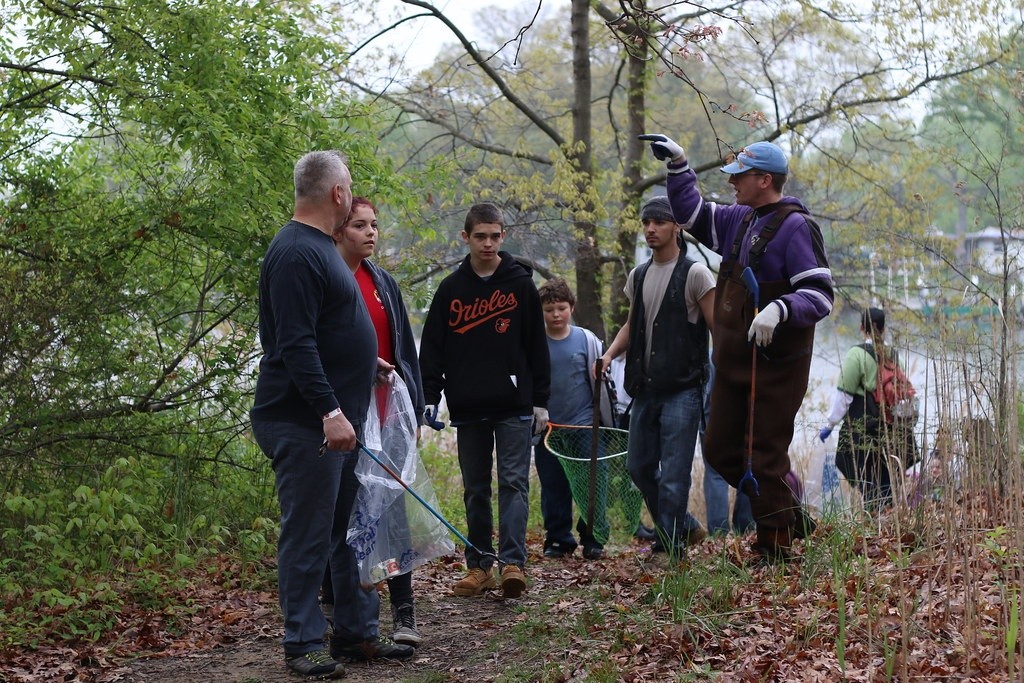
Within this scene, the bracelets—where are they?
[322,408,342,422]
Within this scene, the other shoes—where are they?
[634,524,655,539]
[545,547,562,557]
[584,547,601,557]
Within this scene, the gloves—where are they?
[531,407,549,437]
[748,299,788,347]
[820,428,831,442]
[638,134,683,162]
[423,405,445,431]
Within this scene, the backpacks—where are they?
[852,344,919,428]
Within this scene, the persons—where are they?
[820,308,921,521]
[250,151,415,681]
[419,203,549,598]
[531,277,620,561]
[591,197,754,569]
[320,198,426,646]
[636,134,848,564]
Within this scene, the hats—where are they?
[641,196,675,221]
[720,143,787,174]
[862,308,884,334]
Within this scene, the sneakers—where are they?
[454,567,497,595]
[501,564,526,597]
[286,650,345,680]
[329,635,413,663]
[390,596,419,643]
